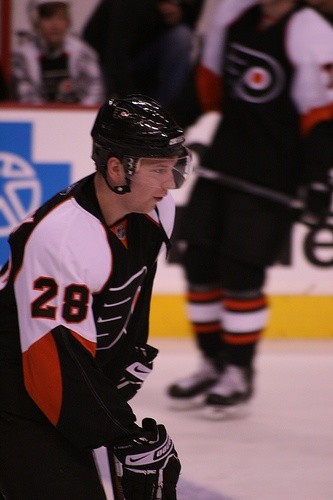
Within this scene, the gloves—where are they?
[111,425,180,500]
[111,344,159,403]
[304,181,331,215]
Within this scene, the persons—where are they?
[0,94,193,500]
[153,0,333,420]
[9,0,212,280]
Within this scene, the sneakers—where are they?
[200,365,256,419]
[166,360,222,411]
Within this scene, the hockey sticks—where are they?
[197,165,333,267]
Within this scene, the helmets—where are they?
[90,94,192,195]
[26,0,73,43]
